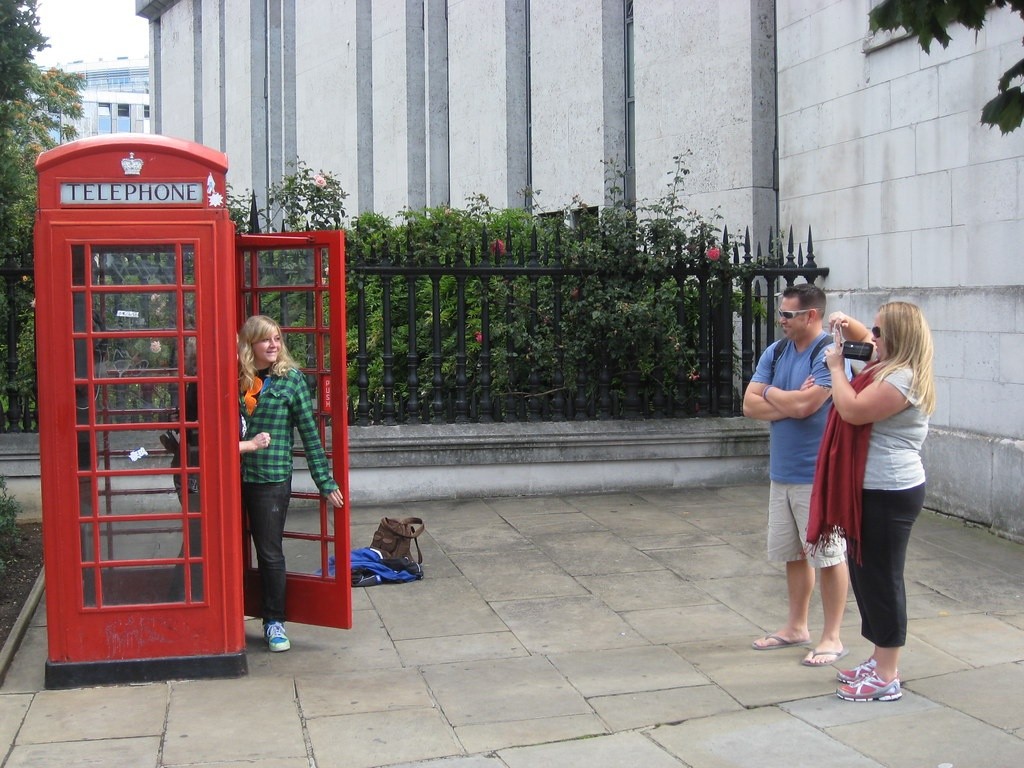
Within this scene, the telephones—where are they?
[72,266,110,444]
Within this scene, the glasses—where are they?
[871,327,881,338]
[778,307,810,319]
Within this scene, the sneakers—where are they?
[835,670,902,701]
[836,657,900,685]
[264,621,290,651]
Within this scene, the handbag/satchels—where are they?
[370,516,425,564]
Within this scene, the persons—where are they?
[743,284,852,667]
[174,316,344,653]
[824,302,933,701]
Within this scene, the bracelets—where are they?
[764,385,772,399]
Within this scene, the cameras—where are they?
[831,323,842,343]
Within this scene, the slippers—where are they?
[752,632,813,651]
[803,640,850,666]
